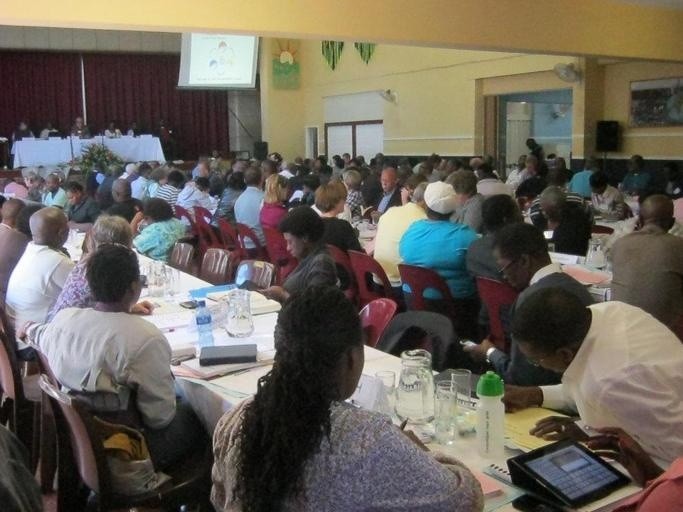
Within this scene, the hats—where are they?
[425,181,459,215]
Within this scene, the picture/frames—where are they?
[629,77,683,130]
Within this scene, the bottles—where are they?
[475,370,506,458]
[195,300,215,349]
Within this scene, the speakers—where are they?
[253,140,270,160]
[595,119,621,152]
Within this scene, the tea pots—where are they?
[147,260,173,297]
[585,239,607,270]
[217,288,254,338]
[396,350,435,422]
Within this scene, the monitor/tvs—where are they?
[507,437,631,508]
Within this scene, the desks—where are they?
[7,133,169,169]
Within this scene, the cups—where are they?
[437,370,473,444]
[375,371,396,416]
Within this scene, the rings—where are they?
[560,424,566,432]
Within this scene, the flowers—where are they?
[55,138,127,181]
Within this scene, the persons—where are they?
[209,285,486,511]
[25,245,205,512]
[585,425,683,511]
[2,109,681,392]
[501,287,682,476]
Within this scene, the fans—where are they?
[550,60,585,86]
[375,87,399,106]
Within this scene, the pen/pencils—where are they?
[400,418,408,432]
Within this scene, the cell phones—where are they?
[180,300,198,309]
[512,493,571,512]
[460,338,478,346]
